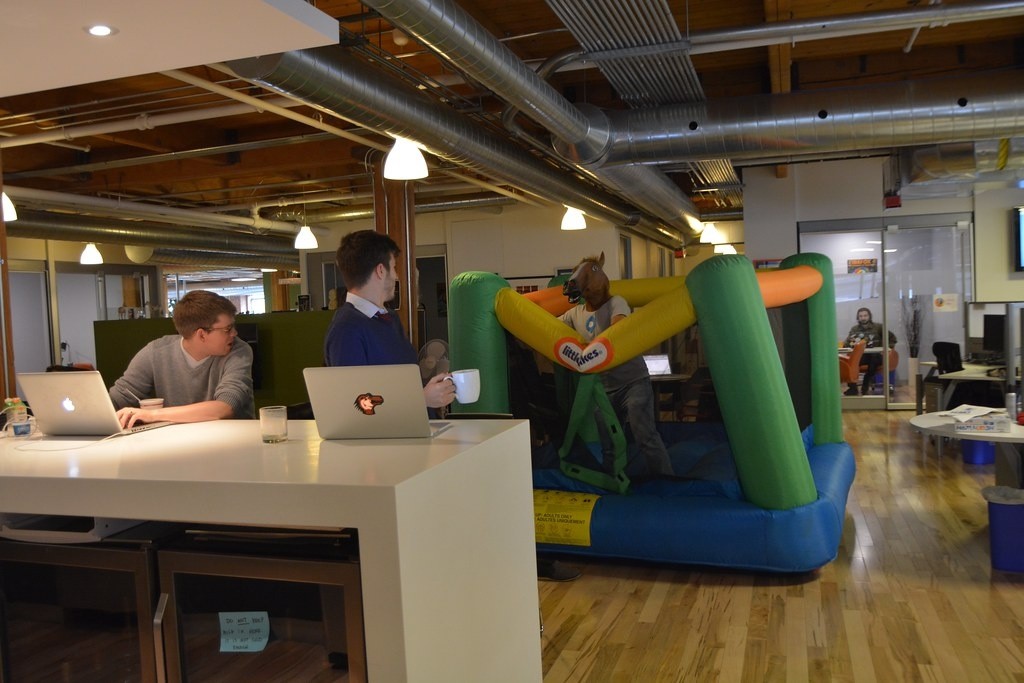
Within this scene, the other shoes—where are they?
[844,389,858,395]
[862,378,868,393]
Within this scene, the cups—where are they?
[139,398,164,410]
[442,369,481,404]
[259,405,288,444]
[119,306,146,320]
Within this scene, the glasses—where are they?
[197,321,235,335]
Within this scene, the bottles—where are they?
[6,397,27,433]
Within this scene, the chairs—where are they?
[838,340,867,384]
[931,342,962,373]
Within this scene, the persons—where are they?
[108,290,256,430]
[540,250,676,477]
[842,308,897,396]
[324,230,456,419]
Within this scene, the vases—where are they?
[908,358,919,396]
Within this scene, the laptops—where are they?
[641,354,692,381]
[15,371,173,436]
[304,365,453,440]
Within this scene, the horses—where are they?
[563,251,610,306]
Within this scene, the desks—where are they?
[0,418,540,683]
[919,362,1023,408]
[840,346,891,394]
[911,409,1024,473]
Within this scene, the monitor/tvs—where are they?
[982,314,1005,352]
[1014,205,1024,272]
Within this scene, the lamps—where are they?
[700,221,721,243]
[561,204,587,232]
[295,197,318,249]
[79,242,104,266]
[3,191,16,223]
[383,135,428,180]
